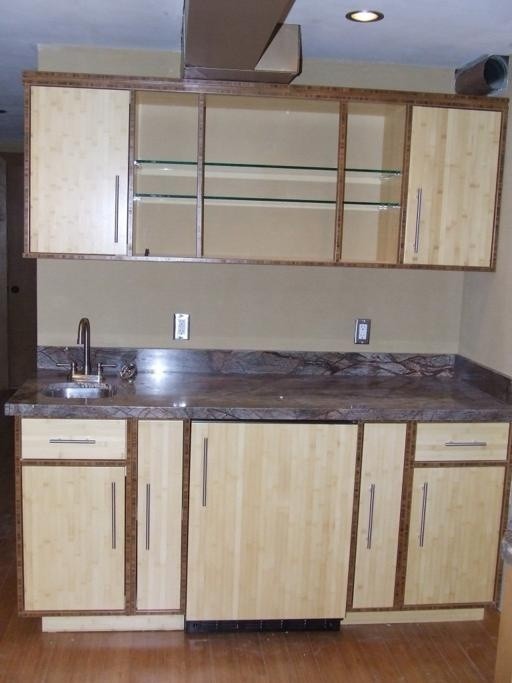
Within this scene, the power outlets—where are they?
[352,317,371,345]
[172,311,190,340]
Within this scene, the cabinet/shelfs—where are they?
[343,413,512,628]
[16,415,183,635]
[185,415,363,626]
[401,97,510,270]
[21,74,128,257]
[134,79,403,267]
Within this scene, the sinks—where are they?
[45,386,110,400]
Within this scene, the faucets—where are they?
[75,316,92,374]
